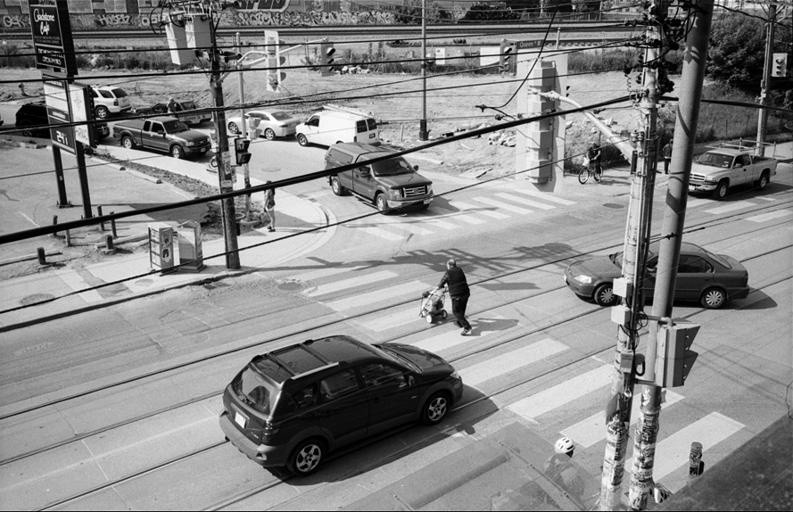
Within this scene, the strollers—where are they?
[418,286,448,323]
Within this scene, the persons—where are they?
[661,138,674,174]
[166,97,177,118]
[436,258,472,336]
[422,291,444,312]
[263,180,276,233]
[584,142,602,180]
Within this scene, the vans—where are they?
[324,142,434,215]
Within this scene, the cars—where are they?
[15,101,111,142]
[89,85,134,120]
[219,334,464,479]
[563,240,749,306]
[226,108,296,141]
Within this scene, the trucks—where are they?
[295,108,379,149]
[686,137,778,199]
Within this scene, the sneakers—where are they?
[267,224,275,232]
[453,319,472,336]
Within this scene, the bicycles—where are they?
[577,156,603,184]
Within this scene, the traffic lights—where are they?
[320,41,337,78]
[498,38,518,78]
[653,324,699,389]
[771,52,788,78]
[264,30,287,92]
[234,136,252,165]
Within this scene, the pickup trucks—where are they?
[113,115,212,159]
[131,99,213,126]
